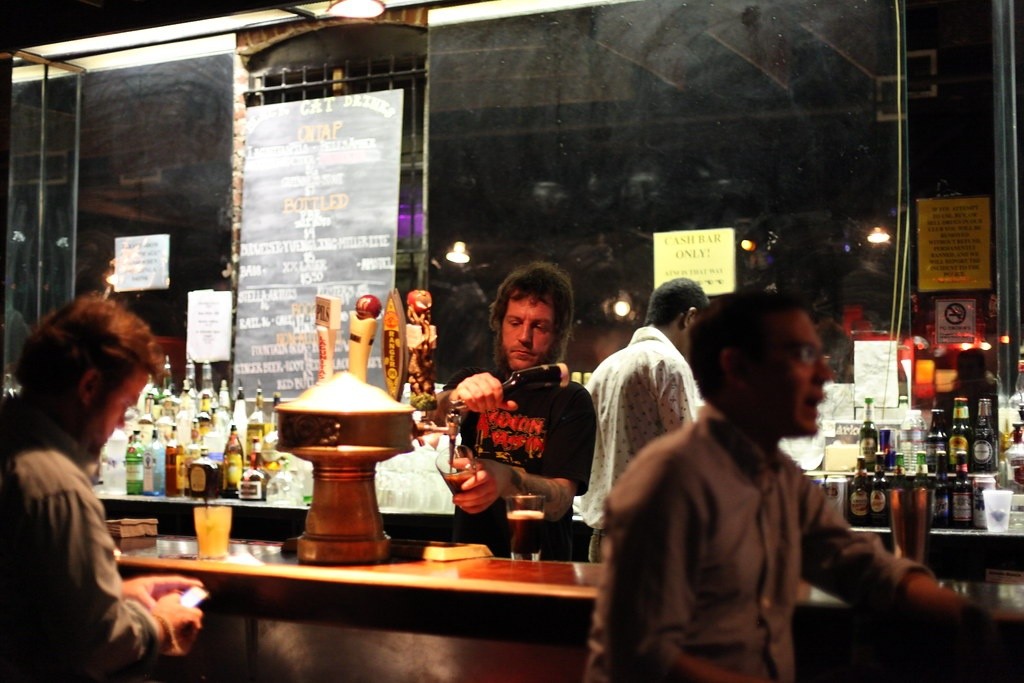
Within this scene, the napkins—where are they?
[106,518,158,538]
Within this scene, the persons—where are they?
[580,290,994,683]
[0,290,204,683]
[588,278,711,562]
[434,261,596,561]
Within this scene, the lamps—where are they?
[447,242,471,264]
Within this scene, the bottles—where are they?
[846,353,1024,529]
[94,358,306,505]
[448,361,570,419]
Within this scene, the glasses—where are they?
[783,345,832,367]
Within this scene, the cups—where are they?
[505,494,546,561]
[982,489,1013,533]
[436,442,479,495]
[194,506,232,559]
